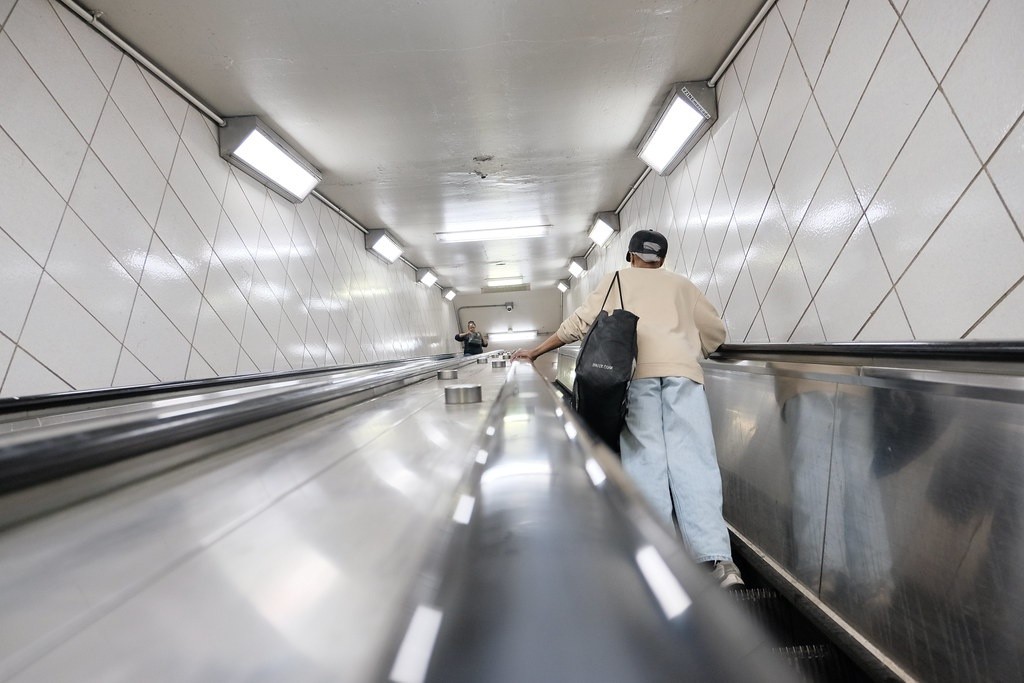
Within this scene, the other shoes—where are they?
[710,560,746,589]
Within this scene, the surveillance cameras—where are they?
[505,302,513,312]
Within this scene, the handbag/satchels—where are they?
[469,333,482,346]
[570,271,640,453]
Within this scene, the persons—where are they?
[454,321,488,357]
[505,228,750,589]
[763,351,898,616]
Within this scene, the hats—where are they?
[625,229,668,263]
